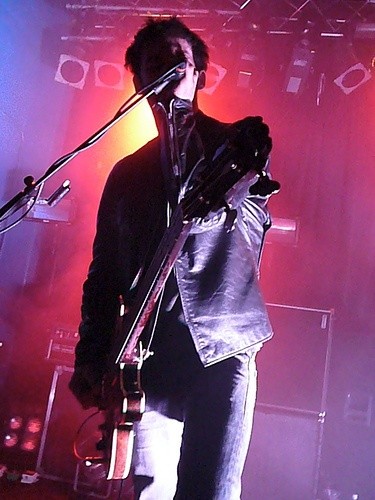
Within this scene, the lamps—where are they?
[54,35,372,106]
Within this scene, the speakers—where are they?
[240,301,334,500]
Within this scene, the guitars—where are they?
[95,116,280,481]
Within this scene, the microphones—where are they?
[174,62,186,81]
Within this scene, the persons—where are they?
[66,17,280,500]
